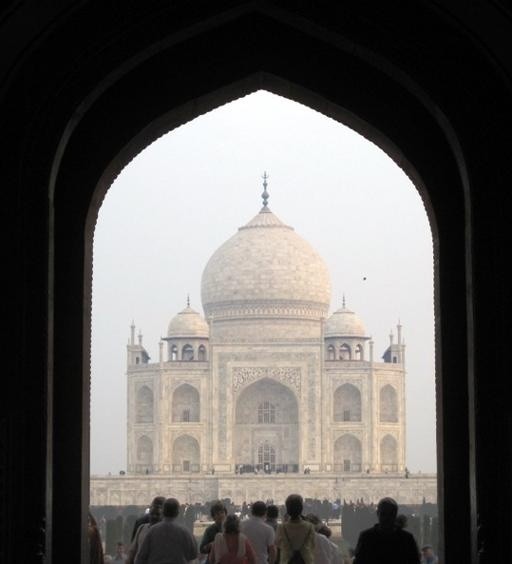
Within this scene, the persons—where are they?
[112,496,197,562]
[200,495,340,563]
[344,497,438,562]
[303,467,311,474]
[235,461,297,474]
[89,516,104,562]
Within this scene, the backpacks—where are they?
[282,524,312,564]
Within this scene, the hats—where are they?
[421,544,433,550]
